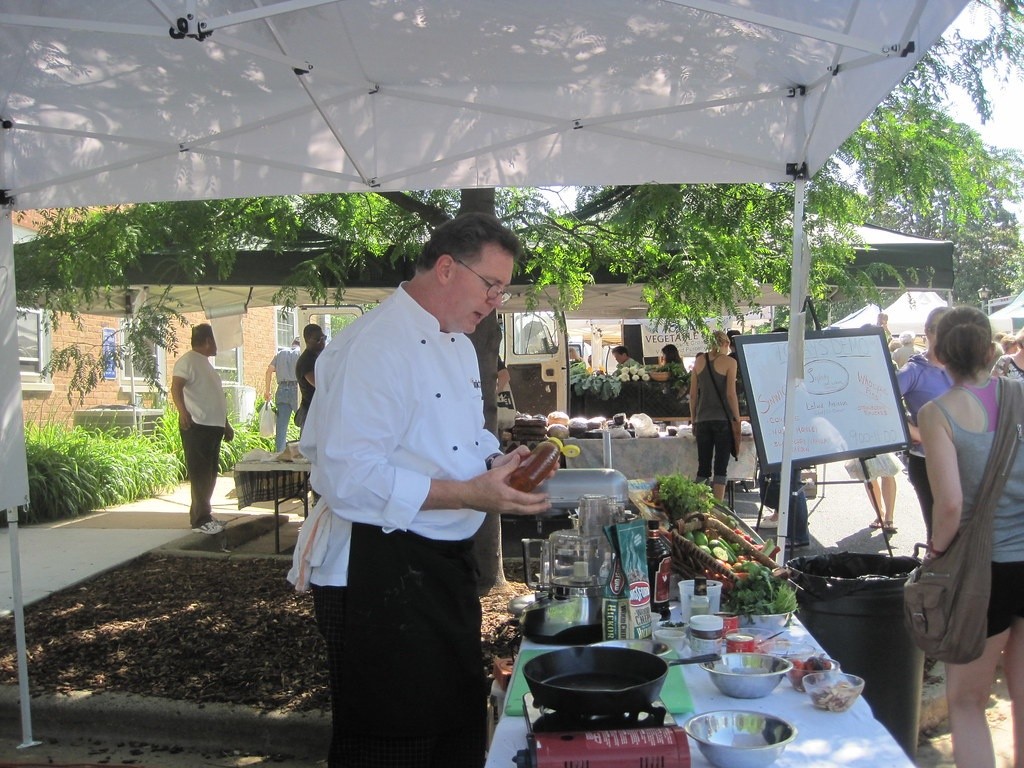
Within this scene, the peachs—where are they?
[790,657,831,691]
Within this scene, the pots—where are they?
[521,646,721,716]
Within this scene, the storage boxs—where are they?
[802,468,818,498]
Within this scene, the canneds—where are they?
[725,636,755,653]
[689,614,724,656]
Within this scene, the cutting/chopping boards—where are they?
[505,647,690,716]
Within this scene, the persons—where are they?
[988,332,1006,373]
[889,330,921,369]
[171,324,234,533]
[297,211,552,768]
[662,345,681,366]
[917,306,1024,768]
[727,330,740,360]
[990,327,1024,382]
[612,346,640,368]
[759,467,811,552]
[264,336,300,452]
[893,307,954,545]
[853,452,905,531]
[496,356,510,395]
[877,313,892,344]
[295,324,327,430]
[1001,335,1018,355]
[690,331,742,502]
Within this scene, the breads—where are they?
[666,425,678,436]
[547,411,630,439]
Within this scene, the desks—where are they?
[562,435,760,514]
[234,460,312,554]
[486,596,916,768]
[651,416,750,425]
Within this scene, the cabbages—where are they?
[616,361,650,382]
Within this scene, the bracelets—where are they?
[930,548,942,555]
[486,455,499,470]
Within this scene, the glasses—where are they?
[448,252,512,305]
[309,335,328,343]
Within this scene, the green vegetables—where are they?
[569,361,620,400]
[652,469,715,520]
[721,574,798,619]
[650,362,692,389]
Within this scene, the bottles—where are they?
[689,576,710,615]
[645,520,672,622]
[510,437,581,493]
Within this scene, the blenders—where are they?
[504,496,635,645]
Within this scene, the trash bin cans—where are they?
[786,542,927,760]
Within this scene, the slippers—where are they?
[884,520,897,530]
[870,518,881,529]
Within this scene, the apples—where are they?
[684,531,747,569]
[734,529,764,550]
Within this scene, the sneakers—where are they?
[192,522,225,534]
[211,517,227,525]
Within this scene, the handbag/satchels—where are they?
[728,419,739,461]
[294,408,302,427]
[258,399,276,437]
[904,521,991,664]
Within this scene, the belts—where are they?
[279,381,297,386]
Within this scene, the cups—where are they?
[650,612,690,652]
[678,580,724,623]
[726,633,754,653]
[714,613,739,639]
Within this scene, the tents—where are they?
[12,202,954,320]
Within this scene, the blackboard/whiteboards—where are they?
[734,327,913,474]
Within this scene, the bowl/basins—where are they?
[726,628,775,646]
[802,672,865,713]
[761,642,817,662]
[735,608,797,628]
[785,656,840,692]
[586,639,672,656]
[699,653,794,699]
[683,709,798,768]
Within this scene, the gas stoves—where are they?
[521,692,691,768]
